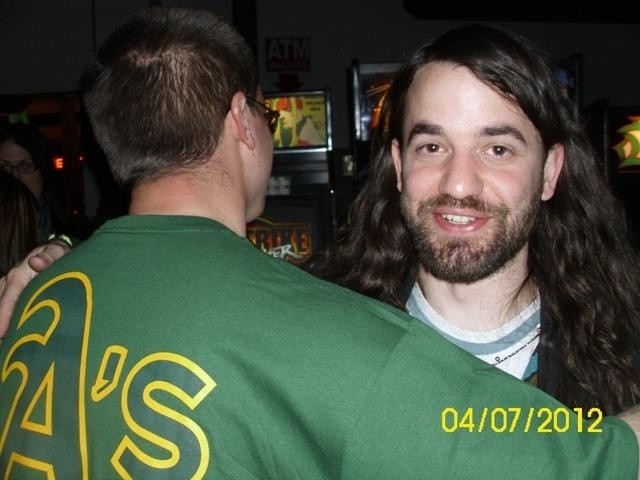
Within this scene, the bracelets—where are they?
[47,229,77,249]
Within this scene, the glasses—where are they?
[3,160,38,176]
[246,93,279,132]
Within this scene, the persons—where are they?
[0,170,44,278]
[0,26,640,416]
[0,121,75,241]
[0,5,639,480]
